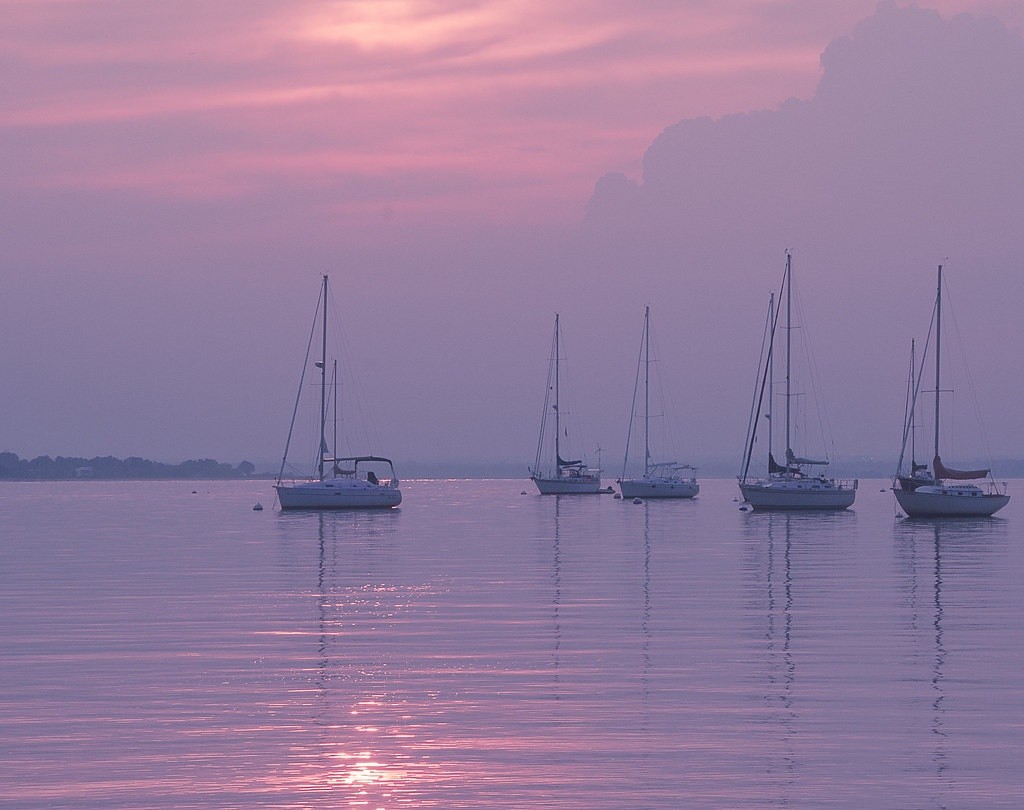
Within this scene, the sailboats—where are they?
[739,251,860,511]
[890,265,1012,520]
[618,304,702,499]
[266,275,401,509]
[895,336,943,492]
[530,311,615,495]
